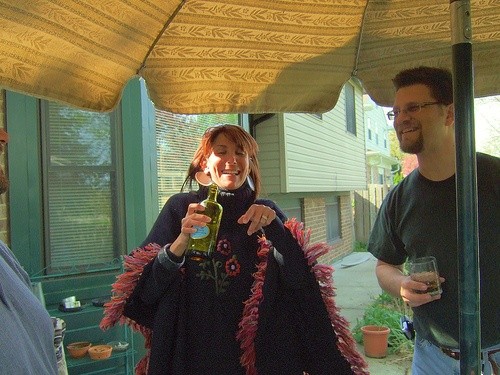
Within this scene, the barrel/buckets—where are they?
[362,325,391,358]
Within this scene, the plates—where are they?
[109,341,130,351]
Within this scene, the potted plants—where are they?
[360,290,391,359]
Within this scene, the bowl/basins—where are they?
[88,346,112,359]
[67,343,90,358]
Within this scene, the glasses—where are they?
[387,102,439,120]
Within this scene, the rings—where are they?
[261,215,267,220]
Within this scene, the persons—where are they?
[367,66,500,375]
[100,124,370,375]
[0,127,58,375]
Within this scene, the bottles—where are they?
[188,185,224,259]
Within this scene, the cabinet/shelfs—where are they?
[48,302,138,375]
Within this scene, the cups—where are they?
[409,257,441,295]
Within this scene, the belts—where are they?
[440,348,500,375]
